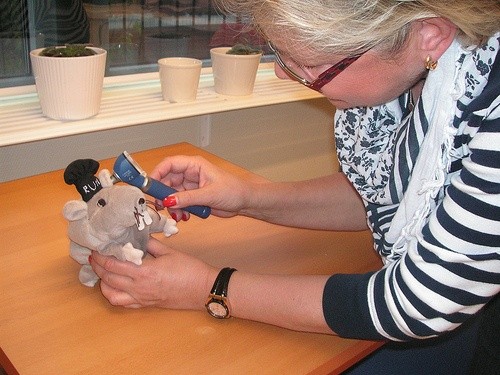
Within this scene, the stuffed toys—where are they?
[63,159,179,288]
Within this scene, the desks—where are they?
[0,142,389,375]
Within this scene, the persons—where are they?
[89,0,500,375]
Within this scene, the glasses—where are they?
[251,14,376,93]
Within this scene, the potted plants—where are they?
[210,43,265,96]
[30,44,107,120]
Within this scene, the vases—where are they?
[157,57,204,104]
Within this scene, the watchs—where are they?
[204,266,237,319]
